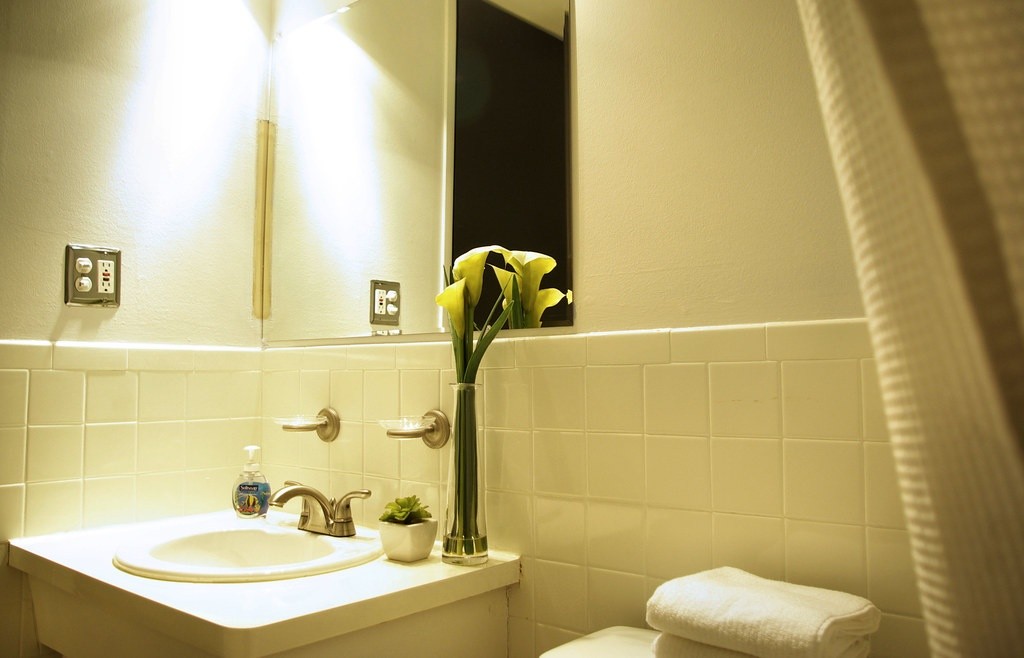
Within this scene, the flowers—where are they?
[437,244,515,560]
[489,241,567,330]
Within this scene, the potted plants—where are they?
[378,495,439,564]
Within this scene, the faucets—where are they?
[267,479,372,537]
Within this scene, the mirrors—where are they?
[258,0,456,345]
[450,0,582,334]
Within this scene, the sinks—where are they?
[111,516,384,583]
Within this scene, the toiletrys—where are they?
[231,444,270,521]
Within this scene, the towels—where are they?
[645,566,882,658]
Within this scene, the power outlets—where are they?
[367,280,403,326]
[64,245,123,309]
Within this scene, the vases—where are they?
[445,383,507,556]
